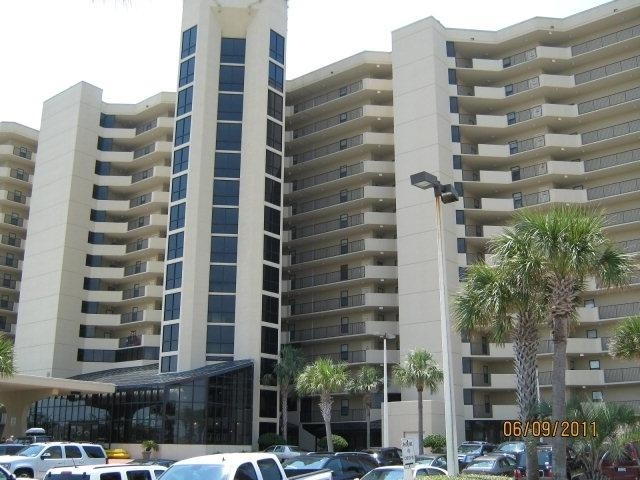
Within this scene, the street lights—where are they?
[411,168,464,474]
[378,333,399,447]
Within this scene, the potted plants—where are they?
[139,438,160,460]
